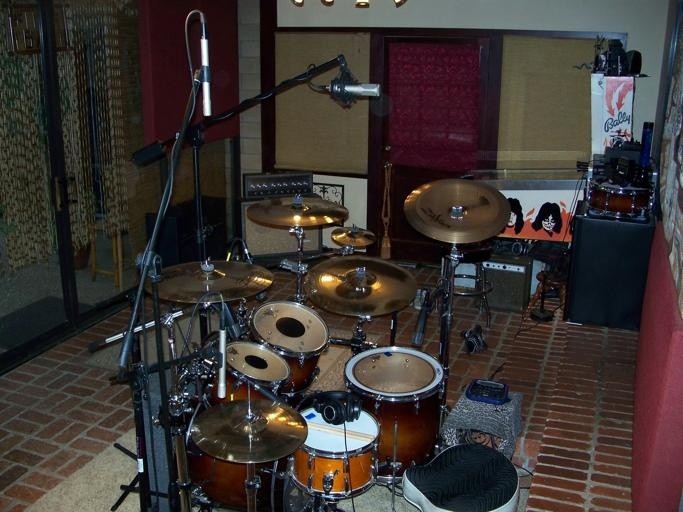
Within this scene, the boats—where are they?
[482,253,533,313]
[626,50,642,75]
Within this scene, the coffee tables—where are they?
[303,253,419,318]
[404,177,512,245]
[332,227,376,247]
[191,398,309,465]
[145,259,276,304]
[246,196,348,229]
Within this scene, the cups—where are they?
[543,218,556,223]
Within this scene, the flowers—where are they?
[322,83,380,96]
[410,295,430,346]
[200,15,212,116]
[218,311,227,398]
[241,240,267,301]
[219,302,241,339]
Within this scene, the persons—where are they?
[532,202,562,234]
[502,197,525,235]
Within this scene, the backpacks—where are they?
[209,341,291,406]
[586,179,653,224]
[343,344,447,484]
[292,404,381,503]
[182,449,277,511]
[249,301,329,394]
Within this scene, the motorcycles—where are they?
[427,277,451,450]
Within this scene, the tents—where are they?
[314,391,361,425]
[509,241,530,257]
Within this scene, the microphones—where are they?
[432,240,494,333]
[448,382,534,477]
